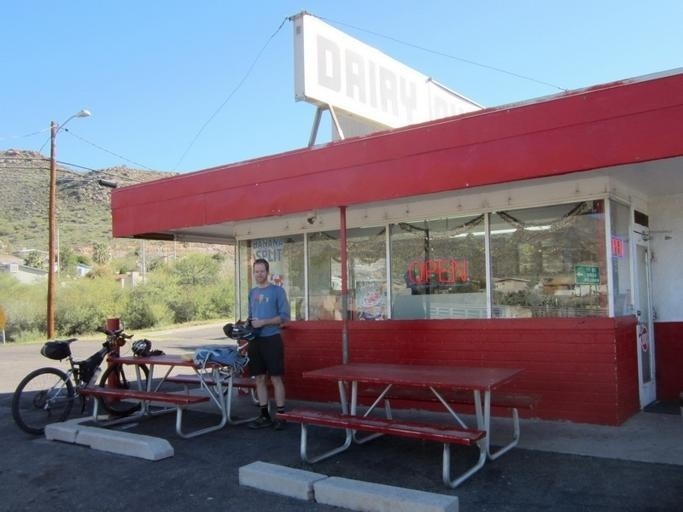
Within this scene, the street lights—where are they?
[47,112,94,344]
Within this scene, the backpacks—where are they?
[192,348,246,370]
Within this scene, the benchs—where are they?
[274,388,544,488]
[78,375,283,438]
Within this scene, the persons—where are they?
[244,258,291,431]
[391,271,429,322]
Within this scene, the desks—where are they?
[302,352,524,488]
[107,354,249,438]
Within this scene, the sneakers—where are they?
[248,415,273,428]
[273,418,286,429]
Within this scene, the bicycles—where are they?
[8,328,156,440]
